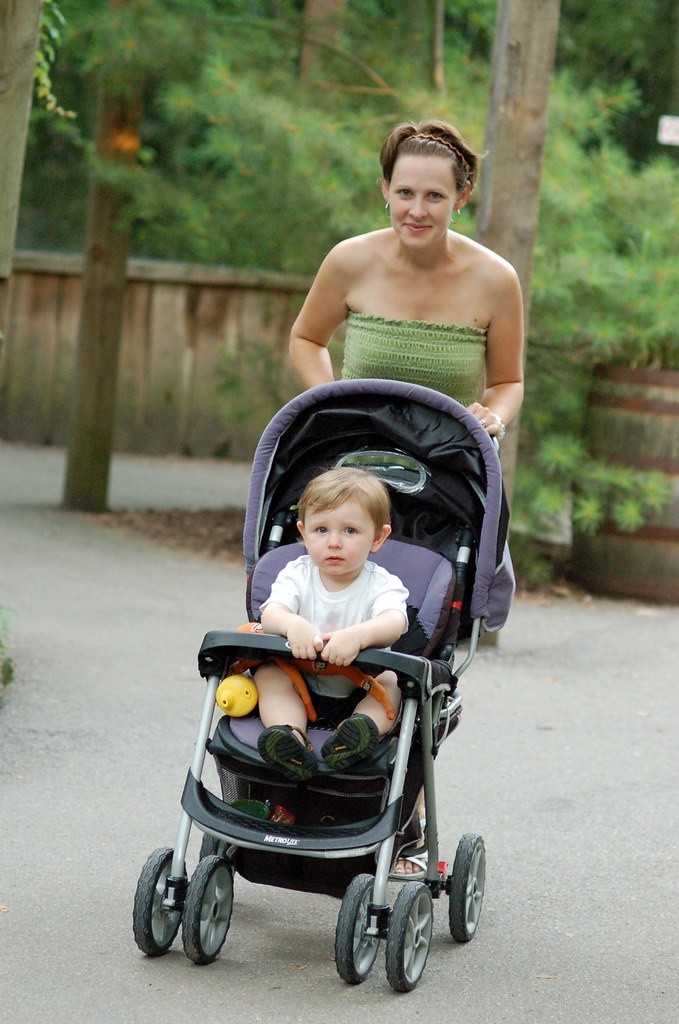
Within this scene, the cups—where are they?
[230,800,269,820]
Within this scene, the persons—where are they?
[239,465,410,787]
[287,119,524,879]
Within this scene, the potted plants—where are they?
[529,232,679,603]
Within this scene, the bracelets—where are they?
[493,415,506,440]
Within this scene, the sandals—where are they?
[390,819,429,880]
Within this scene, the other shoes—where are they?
[321,712,379,770]
[257,725,319,782]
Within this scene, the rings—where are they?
[481,419,486,428]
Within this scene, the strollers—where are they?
[132,378,515,993]
